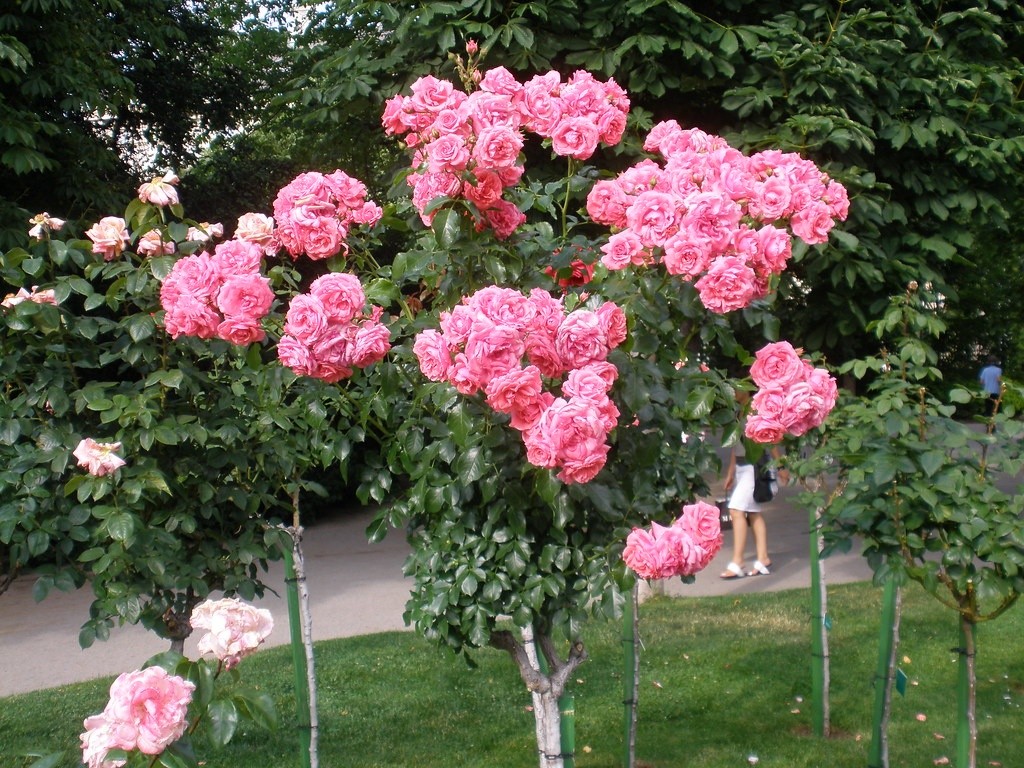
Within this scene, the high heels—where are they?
[746,560,772,576]
[720,561,746,578]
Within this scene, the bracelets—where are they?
[778,466,785,471]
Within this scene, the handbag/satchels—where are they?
[715,486,750,531]
[753,464,773,502]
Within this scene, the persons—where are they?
[980,355,1002,432]
[722,439,787,578]
